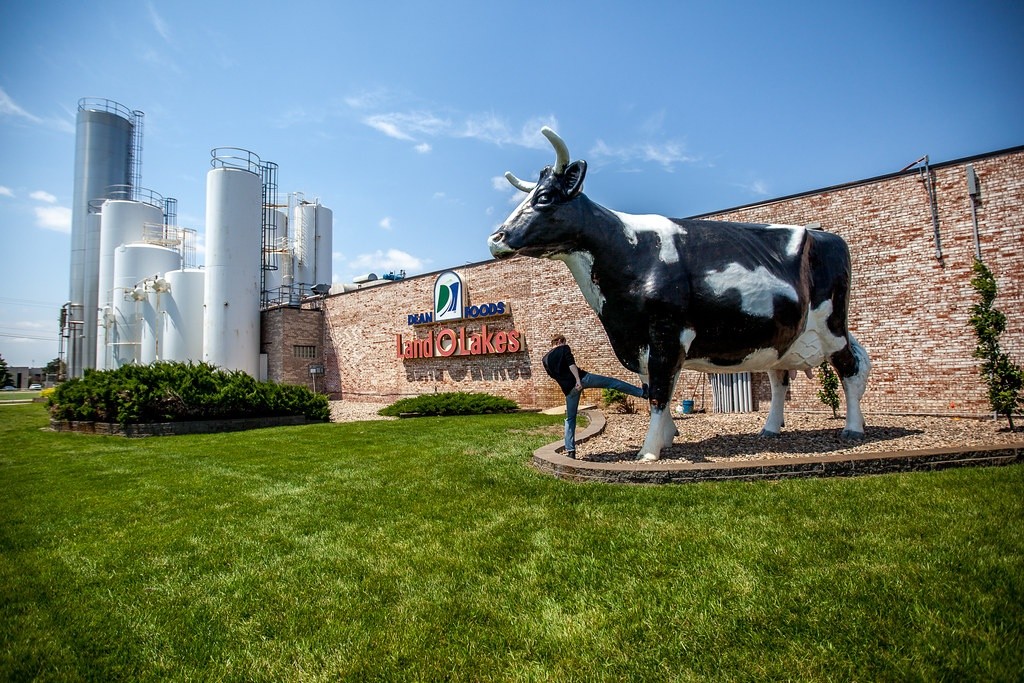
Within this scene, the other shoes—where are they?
[641,384,649,400]
[566,451,576,460]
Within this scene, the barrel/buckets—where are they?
[682,399,694,414]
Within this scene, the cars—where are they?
[3,386,15,390]
[29,384,41,391]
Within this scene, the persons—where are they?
[542,335,649,458]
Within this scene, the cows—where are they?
[487,127,873,461]
